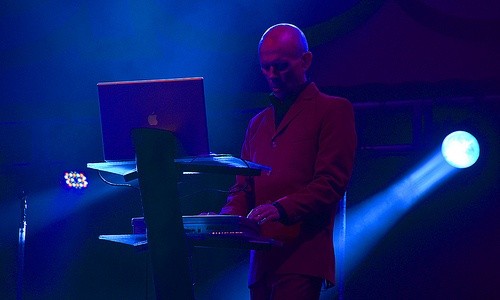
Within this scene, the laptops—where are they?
[97,76,233,163]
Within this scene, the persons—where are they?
[199,22,355,300]
